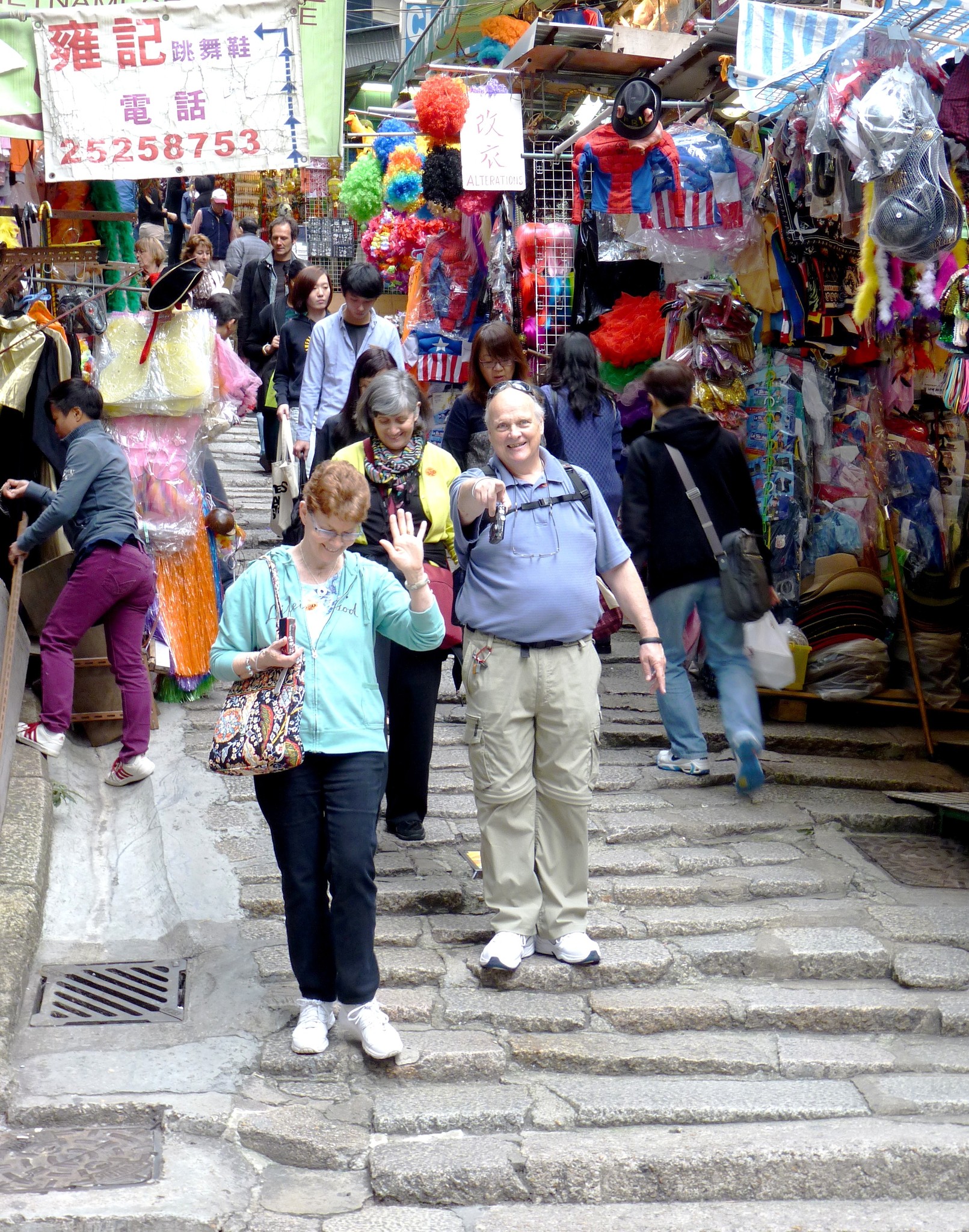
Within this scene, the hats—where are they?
[211,188,228,205]
[794,552,964,653]
[611,76,662,140]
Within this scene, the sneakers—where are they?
[16,721,65,759]
[104,752,155,786]
[656,747,710,775]
[337,996,403,1059]
[291,997,336,1053]
[480,931,534,970]
[730,731,764,798]
[534,931,601,965]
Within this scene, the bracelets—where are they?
[639,637,663,645]
[404,573,430,590]
[246,650,260,679]
[255,648,273,672]
[472,477,501,499]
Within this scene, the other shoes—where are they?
[594,635,613,653]
[260,454,272,473]
[393,819,426,841]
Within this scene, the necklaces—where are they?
[301,539,339,597]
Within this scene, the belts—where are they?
[125,538,149,556]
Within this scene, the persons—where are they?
[448,381,666,966]
[61,176,770,836]
[0,378,160,787]
[208,461,445,1062]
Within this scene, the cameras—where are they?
[279,617,296,654]
[489,504,506,543]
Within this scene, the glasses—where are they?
[134,249,150,253]
[486,380,540,406]
[478,358,513,368]
[512,510,559,557]
[310,513,364,541]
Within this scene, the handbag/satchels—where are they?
[741,610,796,690]
[256,348,279,411]
[592,575,624,639]
[718,528,770,622]
[422,563,462,650]
[269,414,308,546]
[208,553,304,777]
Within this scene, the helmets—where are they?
[855,94,915,176]
